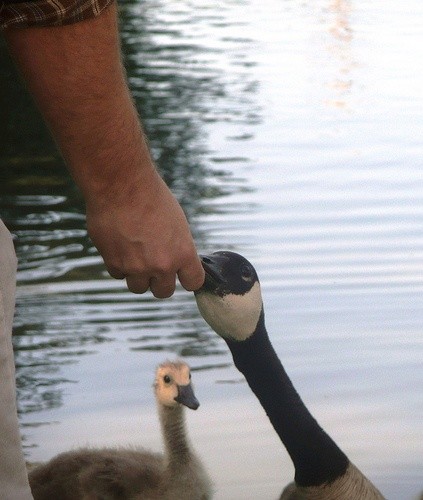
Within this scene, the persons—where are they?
[0,0,208,499]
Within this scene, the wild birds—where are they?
[26,358,215,500]
[195,251,386,500]
